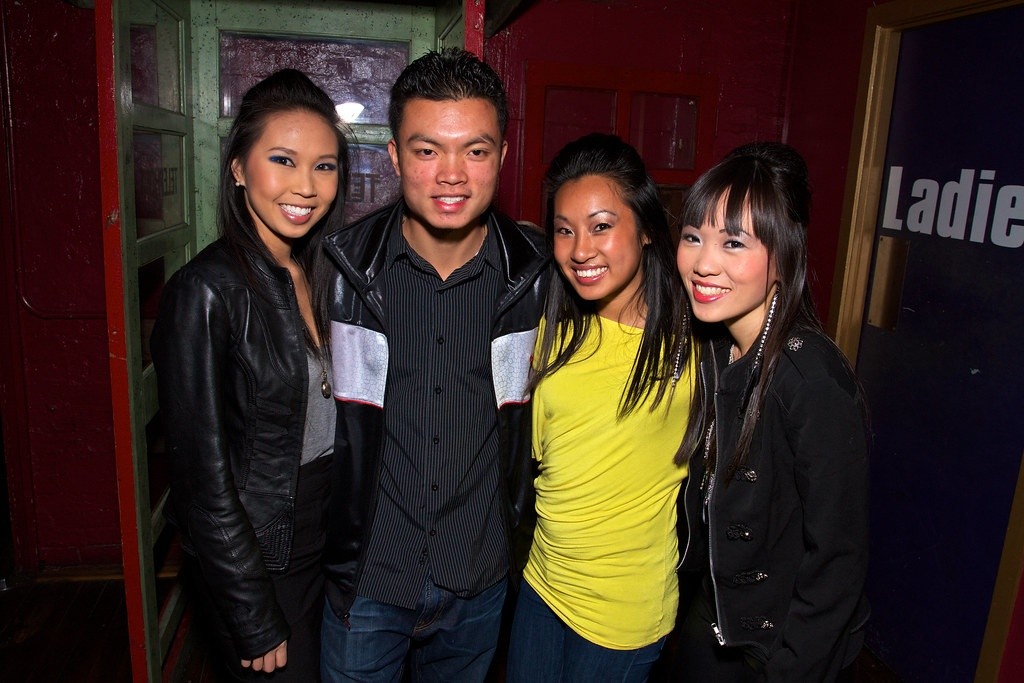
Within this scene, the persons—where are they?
[502,132,702,683]
[149,67,352,683]
[320,46,551,683]
[676,141,872,683]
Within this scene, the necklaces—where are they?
[292,256,331,398]
[728,296,777,367]
